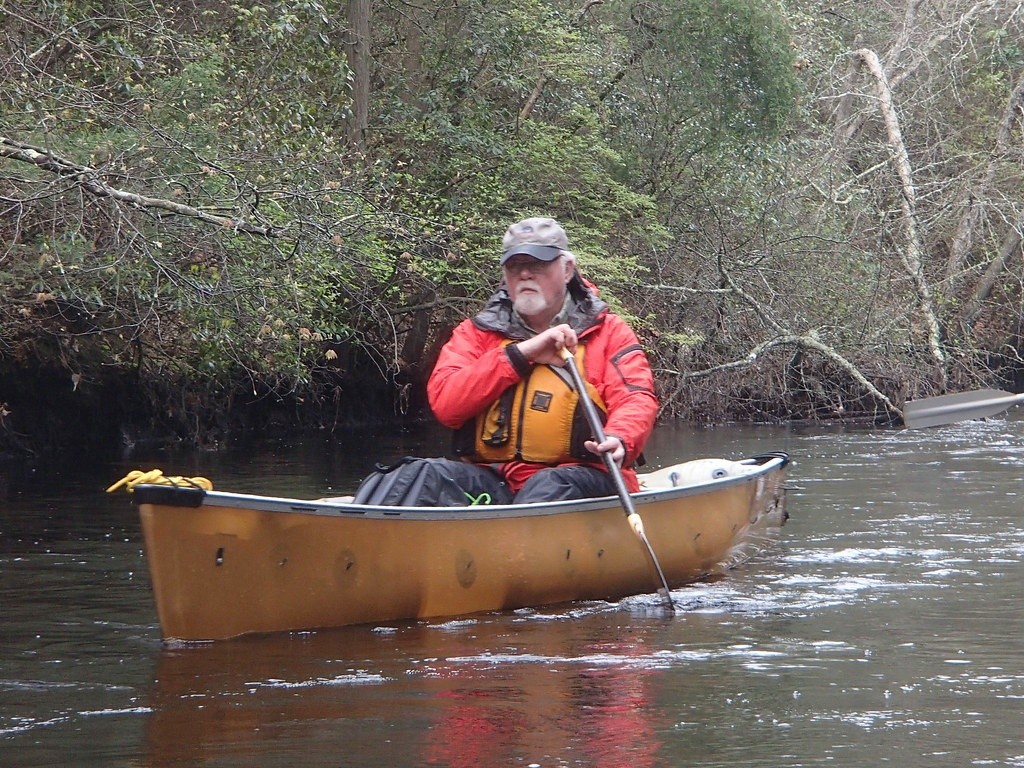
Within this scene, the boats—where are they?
[132,452,793,643]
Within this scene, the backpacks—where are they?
[350,457,439,507]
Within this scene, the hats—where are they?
[499,217,568,265]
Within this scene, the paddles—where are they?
[903,387,1024,431]
[558,344,675,610]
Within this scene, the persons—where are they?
[427,217,658,504]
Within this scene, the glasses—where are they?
[505,257,548,273]
